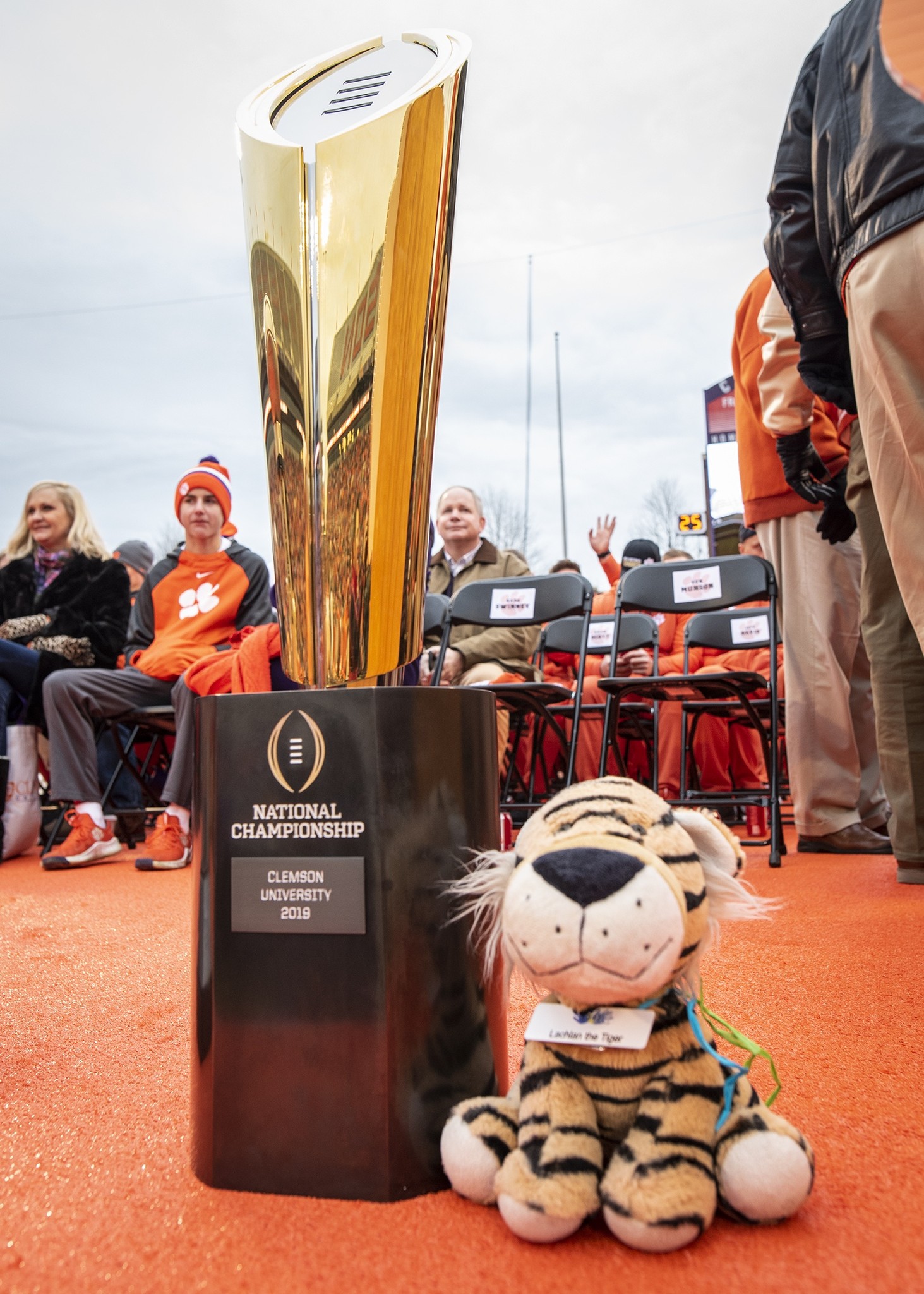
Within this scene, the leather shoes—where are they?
[874,817,892,836]
[797,822,893,854]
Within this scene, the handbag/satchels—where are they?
[2,724,42,860]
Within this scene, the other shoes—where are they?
[896,860,924,883]
[121,808,145,848]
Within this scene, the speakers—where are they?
[189,685,506,1199]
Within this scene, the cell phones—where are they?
[429,652,435,670]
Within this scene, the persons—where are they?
[418,485,542,775]
[567,539,705,811]
[733,266,896,852]
[31,540,155,841]
[41,454,273,869]
[816,395,923,881]
[507,514,622,807]
[0,483,131,860]
[685,523,786,794]
[761,0,924,657]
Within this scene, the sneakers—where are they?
[136,812,190,868]
[41,811,122,868]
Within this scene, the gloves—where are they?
[817,463,858,545]
[775,424,838,504]
[798,332,858,414]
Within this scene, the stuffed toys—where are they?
[440,776,815,1254]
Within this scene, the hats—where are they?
[174,456,232,527]
[115,539,155,575]
[620,539,661,577]
[739,523,757,541]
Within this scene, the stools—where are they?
[40,701,184,857]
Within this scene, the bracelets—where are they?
[599,551,610,558]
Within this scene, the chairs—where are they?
[423,555,794,868]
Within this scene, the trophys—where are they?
[190,28,507,1204]
